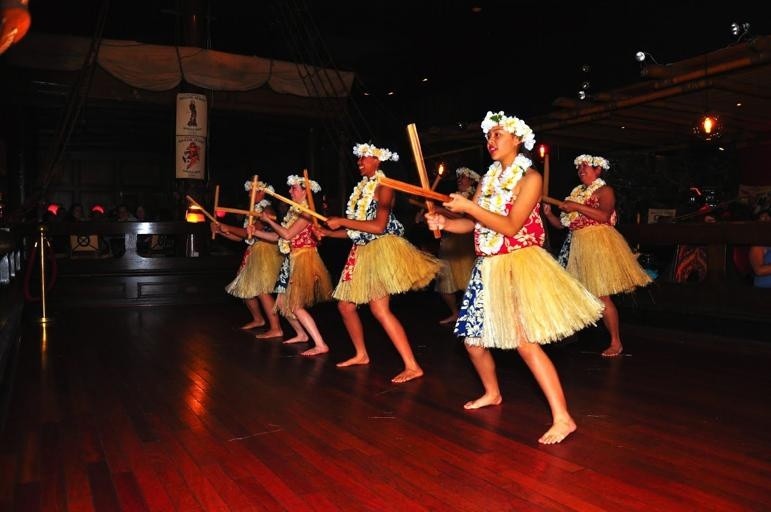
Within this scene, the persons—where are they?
[544,154,624,357]
[311,144,425,383]
[247,174,329,356]
[425,111,579,445]
[210,181,284,339]
[749,211,771,288]
[46,201,159,253]
[416,167,481,324]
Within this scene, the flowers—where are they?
[241,176,322,255]
[340,142,400,239]
[456,110,536,255]
[558,153,610,227]
